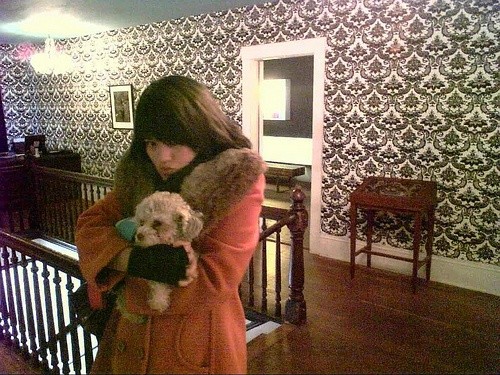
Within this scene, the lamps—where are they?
[29,34,74,76]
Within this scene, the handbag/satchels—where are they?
[68,280,117,337]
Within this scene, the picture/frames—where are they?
[109,83,135,130]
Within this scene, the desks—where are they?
[0,149,81,212]
[348,175,437,295]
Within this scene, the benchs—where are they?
[265,162,306,193]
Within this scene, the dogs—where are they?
[134,192,204,313]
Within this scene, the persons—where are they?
[76,76,268,375]
